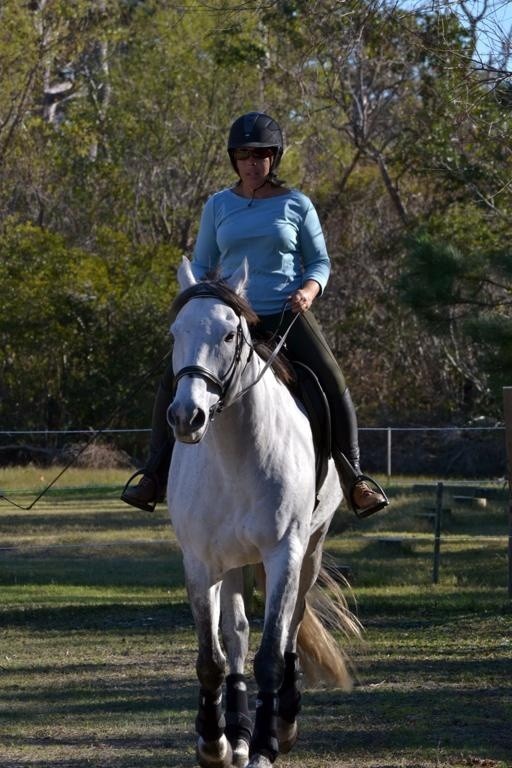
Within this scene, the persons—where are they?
[127,113,385,508]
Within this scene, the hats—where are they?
[227,112,283,151]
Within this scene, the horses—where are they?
[164,255,367,768]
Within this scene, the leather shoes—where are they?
[121,473,165,506]
[347,480,387,512]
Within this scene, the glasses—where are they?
[236,149,270,160]
